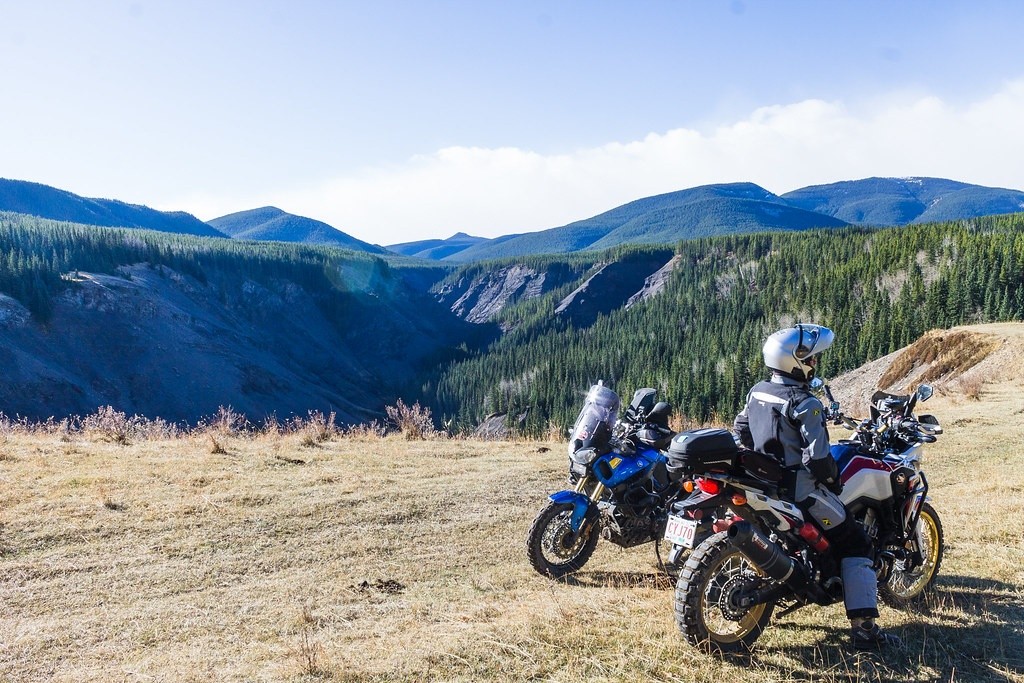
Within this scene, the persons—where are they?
[733,323,900,649]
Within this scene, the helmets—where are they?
[762,328,815,384]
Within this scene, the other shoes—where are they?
[851,623,902,652]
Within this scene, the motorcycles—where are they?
[659,383,946,657]
[523,380,741,581]
